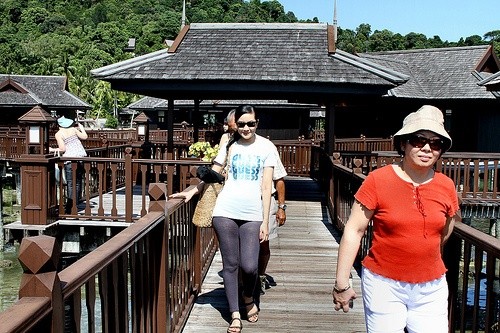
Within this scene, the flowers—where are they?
[188,141,211,158]
[201,144,219,162]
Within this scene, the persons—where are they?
[197,104,288,333]
[332,104,463,333]
[49,114,88,215]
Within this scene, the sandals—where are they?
[244,297,259,323]
[227,318,243,333]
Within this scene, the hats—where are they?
[56,116,74,128]
[392,105,452,153]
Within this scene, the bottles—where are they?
[340,273,353,308]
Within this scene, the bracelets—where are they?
[333,284,350,292]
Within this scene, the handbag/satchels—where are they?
[191,162,233,228]
[52,148,67,185]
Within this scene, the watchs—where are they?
[279,204,287,210]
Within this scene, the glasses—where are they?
[234,121,257,128]
[404,133,445,151]
